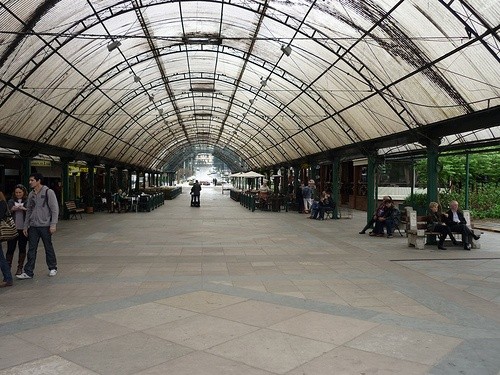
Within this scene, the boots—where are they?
[17,252,27,274]
[6,254,14,268]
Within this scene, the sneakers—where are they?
[49,269,58,276]
[16,273,32,279]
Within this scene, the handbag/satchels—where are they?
[1,204,19,241]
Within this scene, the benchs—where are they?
[406,209,484,250]
[324,201,337,220]
[397,207,413,237]
[64,201,85,220]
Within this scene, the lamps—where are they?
[107,41,122,52]
[242,113,247,117]
[249,99,254,104]
[133,75,142,83]
[261,80,267,87]
[280,44,292,57]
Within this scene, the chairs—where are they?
[240,193,288,212]
[115,192,164,212]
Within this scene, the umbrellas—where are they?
[230,171,264,192]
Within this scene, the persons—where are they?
[303,185,312,214]
[426,203,460,250]
[16,173,59,279]
[310,191,336,220]
[448,201,480,250]
[191,180,201,202]
[214,178,217,186]
[0,192,19,287]
[358,196,401,238]
[6,184,29,276]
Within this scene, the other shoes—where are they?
[437,245,447,250]
[464,246,471,250]
[0,281,13,288]
[387,234,394,238]
[359,231,365,234]
[453,241,460,245]
[474,235,480,240]
